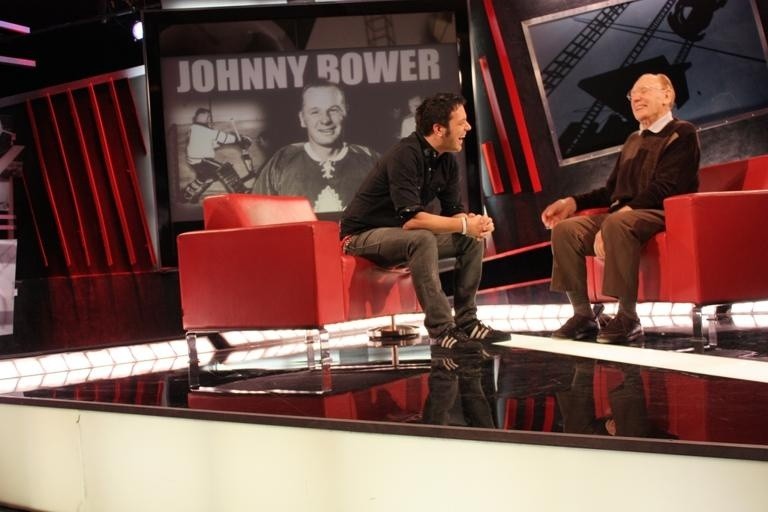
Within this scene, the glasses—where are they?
[626,87,665,101]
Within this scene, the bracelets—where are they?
[462,218,467,234]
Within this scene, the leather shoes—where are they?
[552,341,644,371]
[552,311,644,344]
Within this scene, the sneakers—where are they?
[430,318,511,358]
[431,345,511,372]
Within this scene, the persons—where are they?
[400,92,423,137]
[421,343,511,429]
[563,356,651,438]
[541,73,701,343]
[252,80,382,214]
[339,92,511,353]
[180,108,252,204]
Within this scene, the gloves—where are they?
[240,136,253,148]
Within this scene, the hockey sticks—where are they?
[229,117,255,186]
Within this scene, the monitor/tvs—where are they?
[140,0,484,274]
[520,0,768,166]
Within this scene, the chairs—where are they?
[566,155,768,340]
[177,194,424,387]
